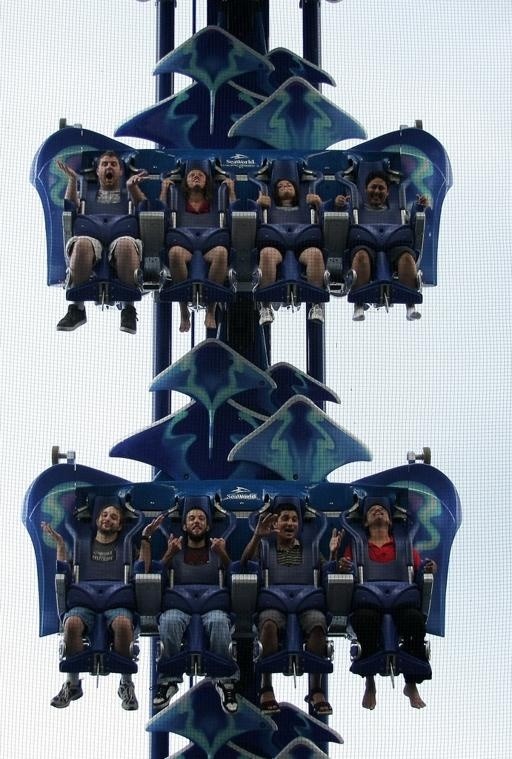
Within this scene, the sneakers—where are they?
[308,304,324,324]
[120,305,136,333]
[152,684,178,708]
[118,680,138,710]
[259,307,274,326]
[50,680,81,708]
[216,682,238,714]
[57,303,86,330]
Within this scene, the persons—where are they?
[148,506,240,716]
[56,150,147,335]
[240,503,346,716]
[43,505,165,710]
[338,502,437,709]
[255,176,326,325]
[334,172,429,321]
[159,168,237,331]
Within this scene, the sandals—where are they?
[306,689,333,714]
[258,686,280,715]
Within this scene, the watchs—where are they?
[141,535,153,544]
